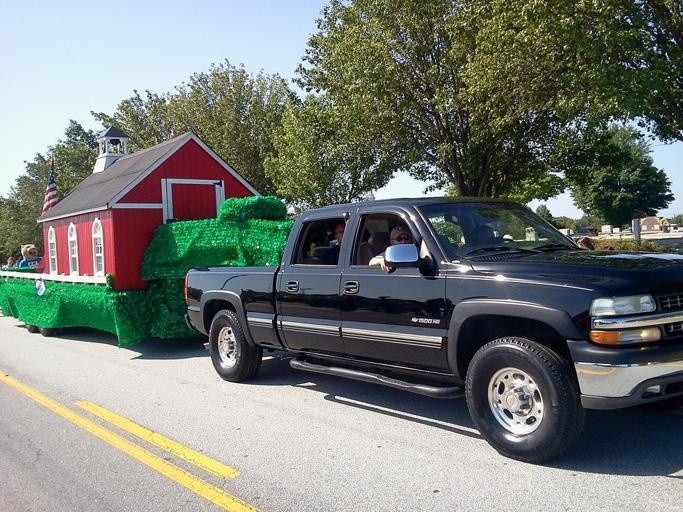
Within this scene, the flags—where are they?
[41,157,58,216]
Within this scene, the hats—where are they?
[390,226,411,239]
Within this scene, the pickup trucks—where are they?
[182,196,683,464]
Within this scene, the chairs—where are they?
[367,232,392,257]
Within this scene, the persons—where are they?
[319,224,344,265]
[575,238,594,250]
[1,244,42,269]
[368,226,412,272]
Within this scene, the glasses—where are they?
[392,236,410,241]
